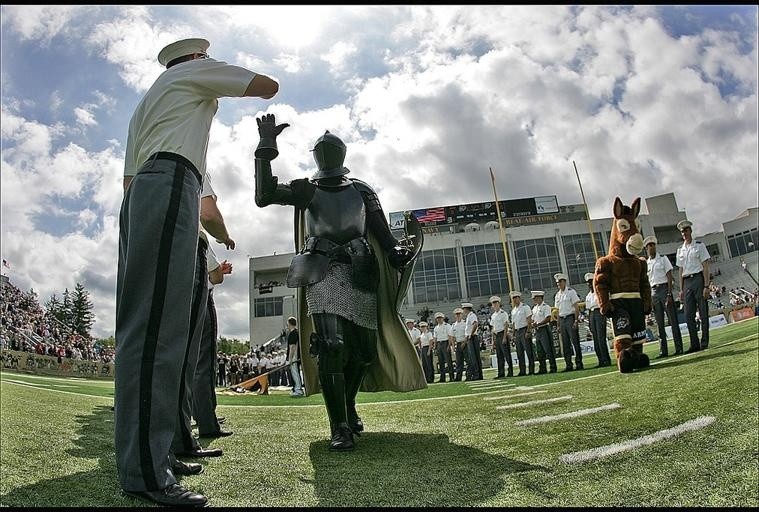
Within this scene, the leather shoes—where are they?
[194,413,233,437]
[593,362,613,369]
[655,349,683,358]
[116,437,222,506]
[685,344,708,354]
[425,374,483,383]
[493,361,584,379]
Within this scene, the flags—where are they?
[3,259,11,269]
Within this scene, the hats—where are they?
[489,295,502,303]
[554,272,569,283]
[676,219,692,231]
[218,350,289,360]
[509,290,521,299]
[584,271,594,282]
[157,38,209,67]
[405,302,474,327]
[642,235,657,248]
[531,290,545,299]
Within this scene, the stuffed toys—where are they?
[594,197,653,374]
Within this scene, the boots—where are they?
[319,367,369,452]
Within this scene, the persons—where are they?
[402,270,613,383]
[643,238,682,359]
[213,316,303,398]
[673,219,712,352]
[1,282,115,380]
[704,254,758,311]
[192,229,235,443]
[253,111,422,454]
[171,169,237,462]
[113,38,280,511]
[748,239,754,253]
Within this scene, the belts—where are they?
[142,152,204,186]
[560,313,574,319]
[589,306,600,311]
[682,270,702,279]
[651,282,668,288]
[514,325,528,331]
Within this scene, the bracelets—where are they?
[666,293,672,297]
